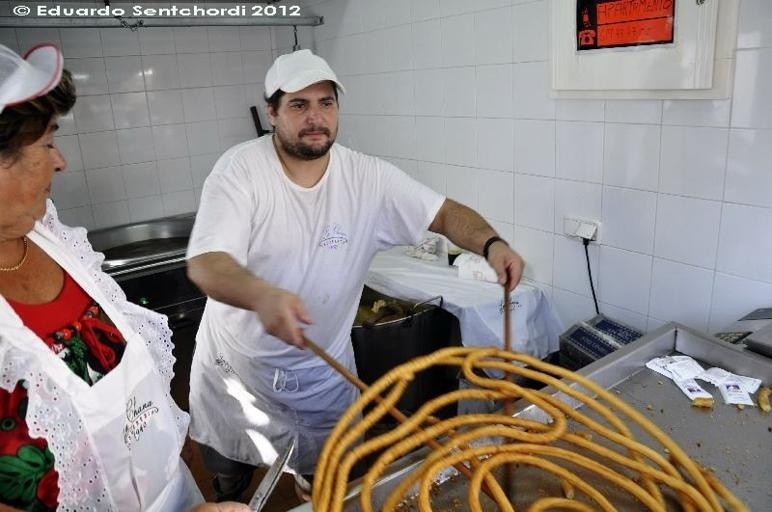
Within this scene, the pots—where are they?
[351,284,464,449]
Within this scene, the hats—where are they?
[264,49,345,99]
[0,43,64,115]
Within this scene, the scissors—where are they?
[247,438,296,512]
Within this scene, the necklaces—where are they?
[1,237,29,272]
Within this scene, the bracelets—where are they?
[483,236,509,260]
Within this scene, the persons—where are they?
[1,39,247,512]
[183,46,526,505]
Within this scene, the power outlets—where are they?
[562,214,580,238]
[575,219,601,242]
